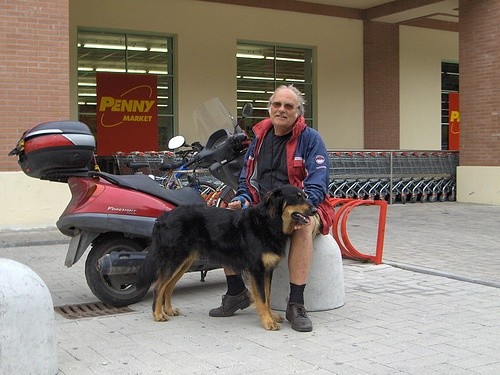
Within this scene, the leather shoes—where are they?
[209,289,254,317]
[285,299,313,332]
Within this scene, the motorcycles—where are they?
[14,94,255,308]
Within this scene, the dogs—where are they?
[132,184,318,331]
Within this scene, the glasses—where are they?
[270,102,298,110]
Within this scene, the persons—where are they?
[209,83,336,332]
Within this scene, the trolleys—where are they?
[112,144,457,204]
